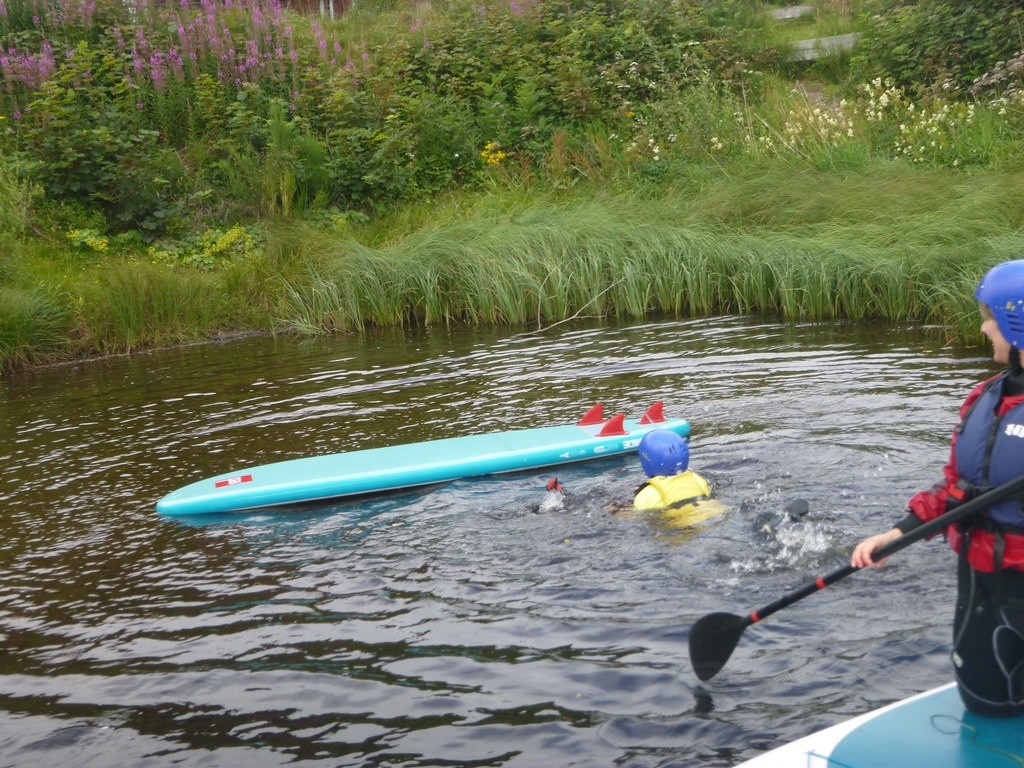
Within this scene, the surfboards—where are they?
[724,680,1023,768]
[154,403,691,528]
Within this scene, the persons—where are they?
[631,428,731,530]
[850,258,1024,715]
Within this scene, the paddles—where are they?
[690,477,1024,683]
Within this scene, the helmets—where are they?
[972,259,1024,349]
[638,429,689,478]
